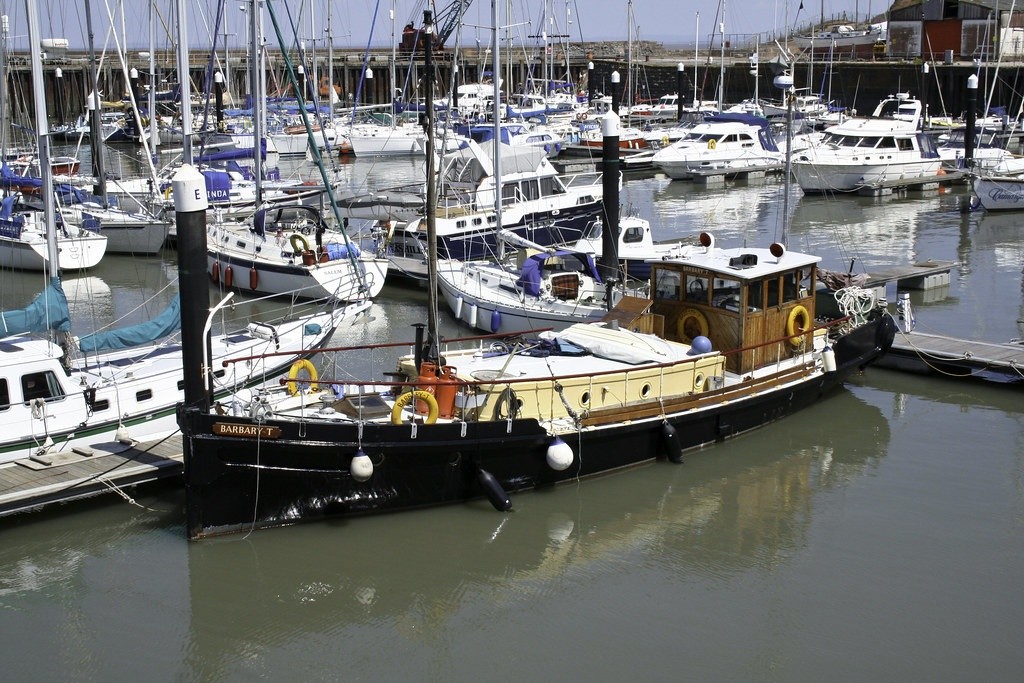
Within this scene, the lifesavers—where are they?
[662,135,669,146]
[478,113,486,121]
[708,138,717,149]
[677,307,711,345]
[576,113,581,120]
[289,234,310,252]
[391,391,437,424]
[787,306,812,346]
[582,113,588,119]
[288,358,320,397]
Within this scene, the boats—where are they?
[2,1,1024,527]
